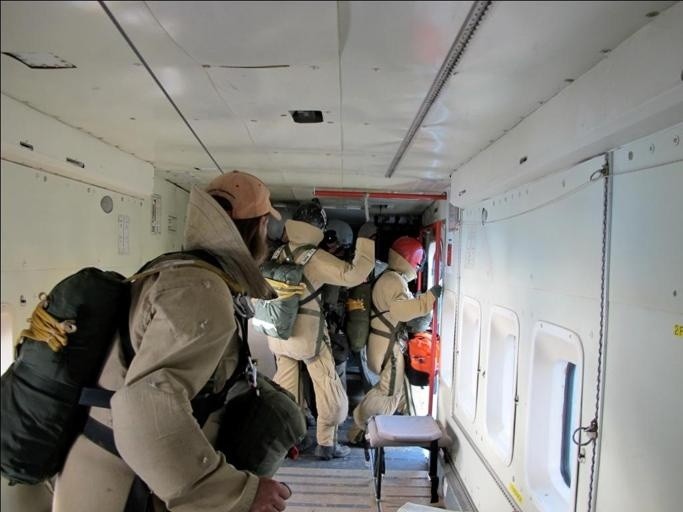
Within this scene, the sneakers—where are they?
[314,444,351,461]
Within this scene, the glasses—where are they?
[325,234,336,244]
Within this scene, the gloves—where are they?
[429,284,441,298]
[356,222,377,240]
[250,473,290,511]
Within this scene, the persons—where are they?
[48,170,293,510]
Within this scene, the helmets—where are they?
[325,218,353,249]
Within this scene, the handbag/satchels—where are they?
[216,370,309,478]
[402,331,441,386]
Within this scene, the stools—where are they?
[368,416,444,512]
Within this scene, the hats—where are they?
[205,170,282,223]
[390,236,426,273]
[292,198,327,232]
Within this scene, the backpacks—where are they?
[347,279,371,349]
[242,243,318,340]
[0,248,249,486]
